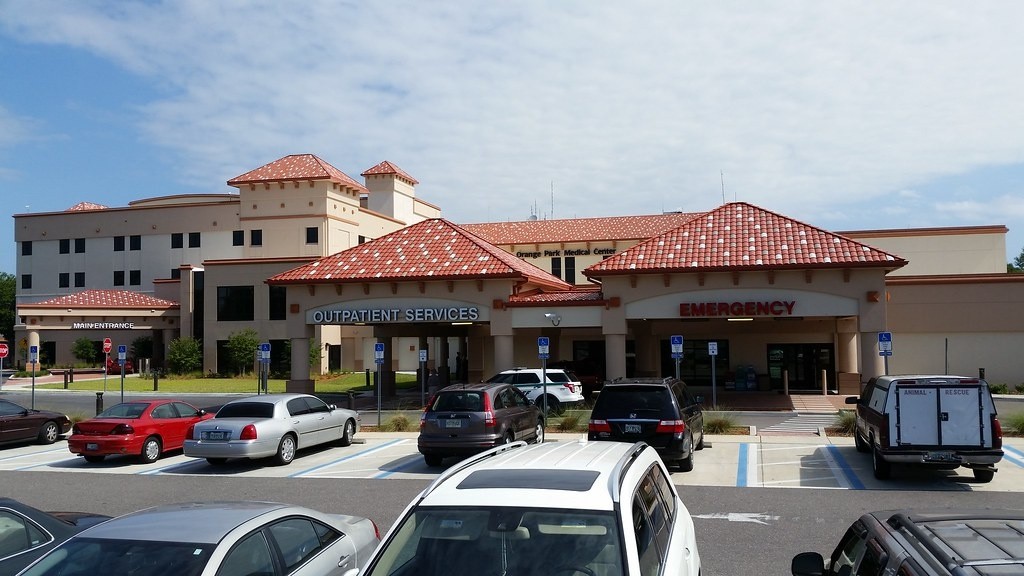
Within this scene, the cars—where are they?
[109,357,134,376]
[180,392,360,468]
[0,400,72,449]
[68,396,216,464]
[16,499,383,576]
[0,496,118,576]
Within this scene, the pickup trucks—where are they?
[845,371,1005,481]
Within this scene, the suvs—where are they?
[465,367,587,418]
[586,375,708,468]
[416,381,545,468]
[790,505,1024,576]
[358,439,703,576]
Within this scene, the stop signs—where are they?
[103,338,113,353]
[0,344,9,360]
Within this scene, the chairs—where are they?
[557,524,620,576]
